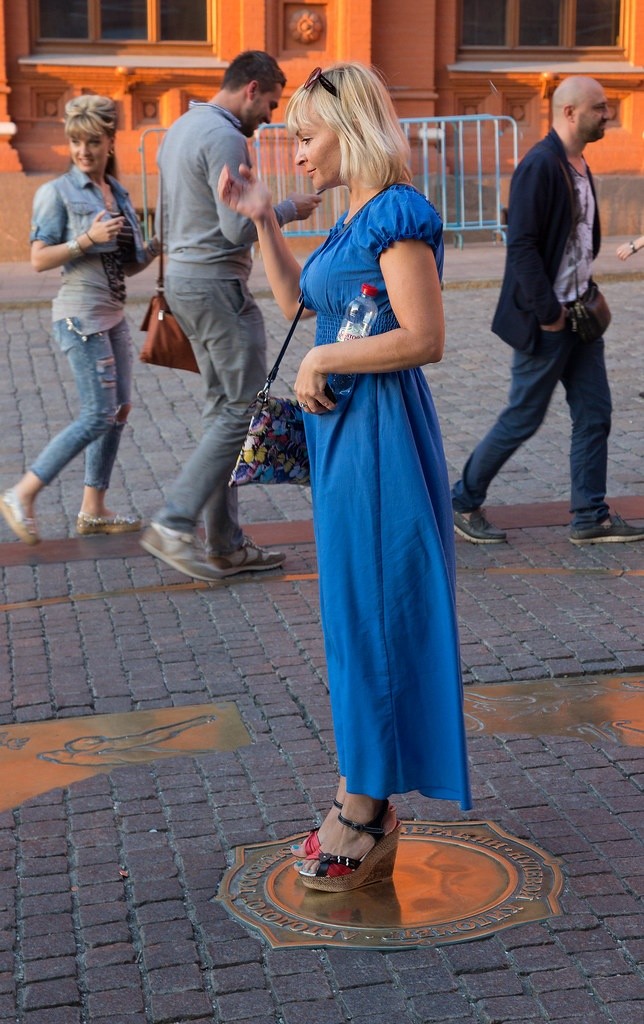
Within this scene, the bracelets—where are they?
[66,239,85,258]
[147,242,160,257]
[629,242,637,252]
[85,231,98,245]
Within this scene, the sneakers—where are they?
[452,509,506,544]
[207,534,285,579]
[570,511,644,544]
[138,522,221,581]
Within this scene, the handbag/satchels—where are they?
[226,396,313,487]
[569,289,611,336]
[137,294,202,374]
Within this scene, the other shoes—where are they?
[76,509,140,535]
[0,487,41,545]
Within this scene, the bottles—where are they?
[327,283,378,400]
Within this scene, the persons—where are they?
[0,95,162,548]
[617,236,644,260]
[218,63,473,892]
[451,75,644,544]
[139,51,323,589]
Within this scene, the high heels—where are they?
[298,799,402,892]
[289,797,342,861]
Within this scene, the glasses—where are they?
[302,65,344,100]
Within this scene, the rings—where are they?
[300,402,308,408]
